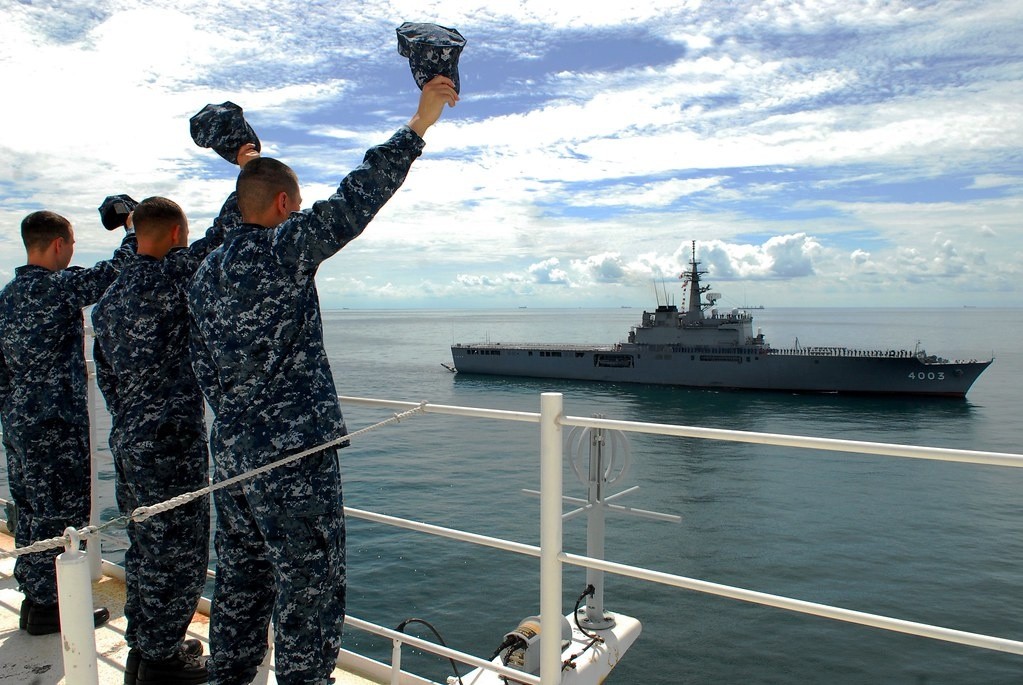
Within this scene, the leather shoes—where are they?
[19,600,31,629]
[25,606,112,635]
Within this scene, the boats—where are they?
[450,239,993,399]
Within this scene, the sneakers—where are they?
[124,638,203,685]
[138,647,212,685]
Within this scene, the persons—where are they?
[91,143,261,685]
[0,210,139,636]
[185,75,460,685]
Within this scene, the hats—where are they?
[99,194,140,230]
[395,21,467,95]
[189,102,261,165]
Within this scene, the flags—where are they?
[678,271,684,279]
[682,274,688,287]
